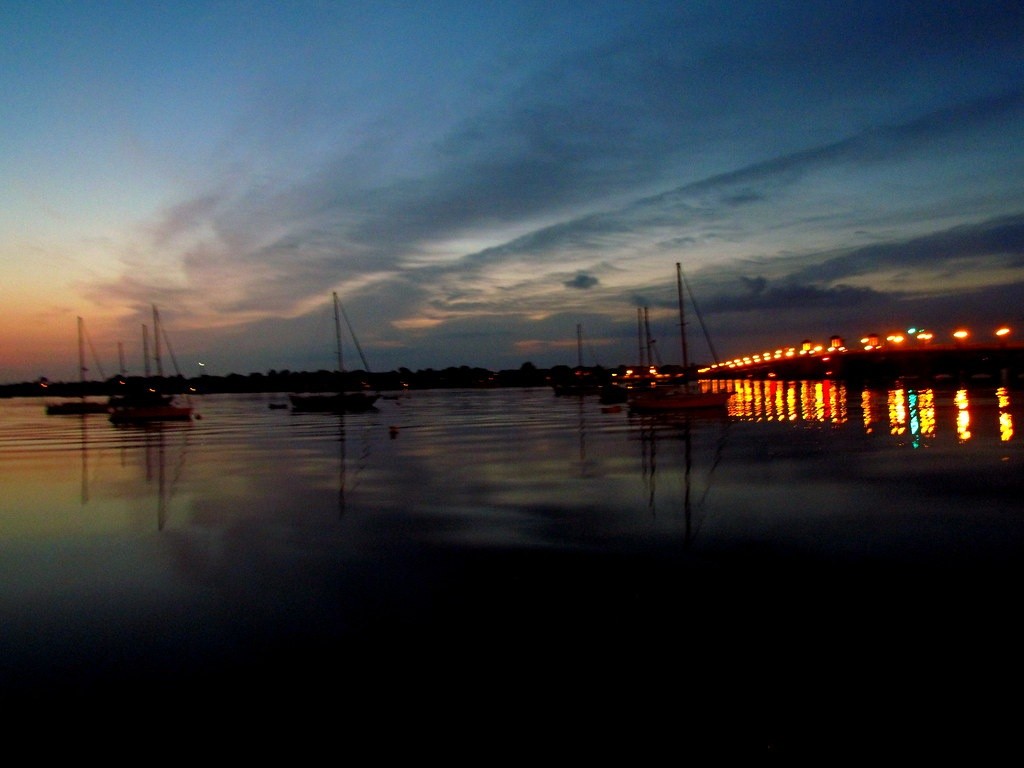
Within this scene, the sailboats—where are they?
[108,304,195,423]
[596,306,675,401]
[289,291,382,412]
[626,262,738,411]
[106,324,173,407]
[45,316,110,415]
[548,323,605,395]
